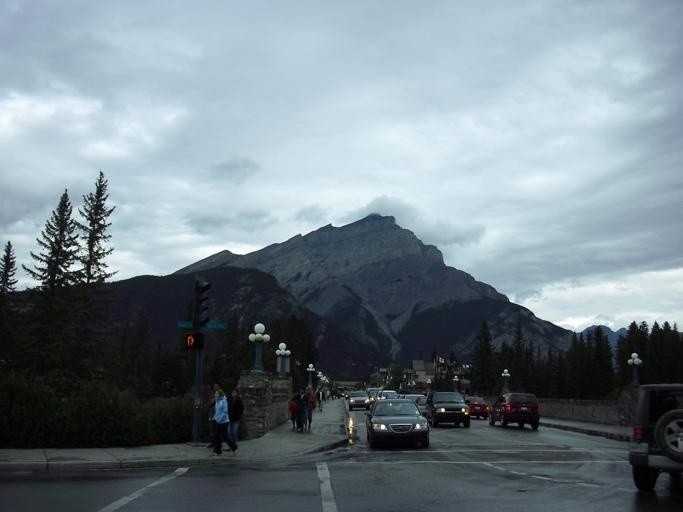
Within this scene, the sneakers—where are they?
[210,446,238,458]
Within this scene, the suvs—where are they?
[628,383,682,490]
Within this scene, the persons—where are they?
[288,383,366,434]
[208,383,245,458]
[420,386,428,397]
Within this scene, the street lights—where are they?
[425,378,431,391]
[452,375,459,391]
[273,343,290,373]
[500,368,511,392]
[382,375,417,392]
[306,362,330,393]
[626,352,643,382]
[247,321,270,372]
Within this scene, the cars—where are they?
[363,398,430,451]
[486,392,541,432]
[348,387,491,430]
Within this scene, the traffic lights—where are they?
[185,332,200,349]
[193,280,210,326]
